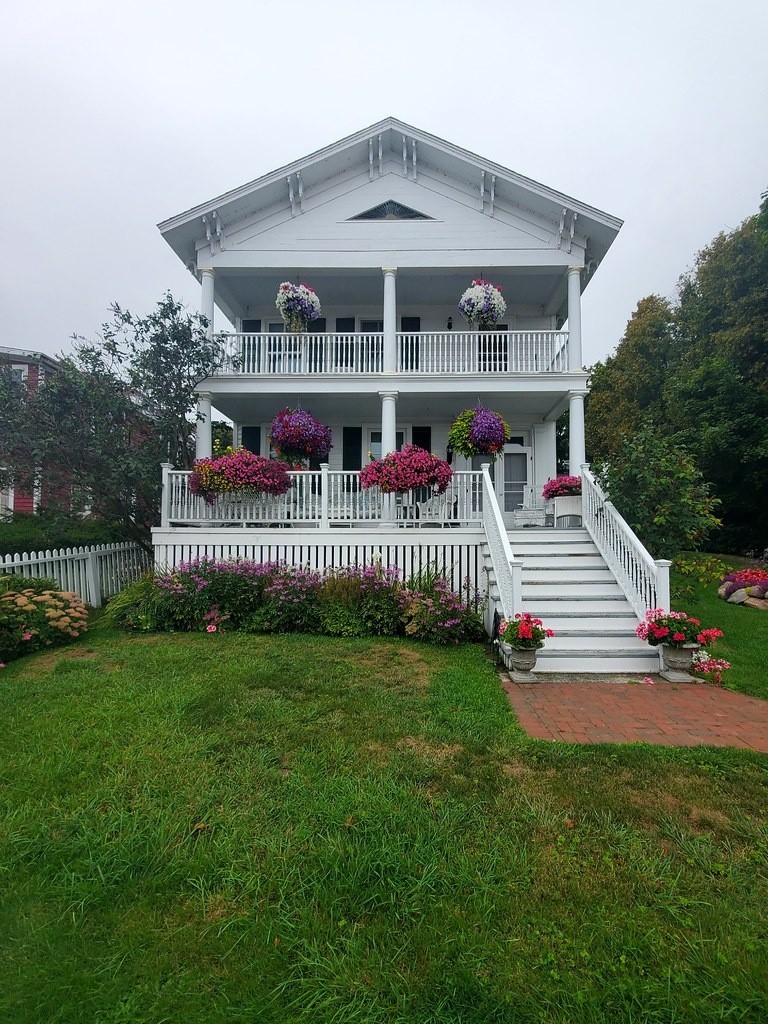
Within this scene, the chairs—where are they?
[417,491,457,529]
[220,485,382,528]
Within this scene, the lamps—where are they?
[445,444,454,464]
[446,316,453,331]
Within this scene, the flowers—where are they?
[187,439,294,508]
[456,279,508,330]
[359,444,455,498]
[635,608,724,652]
[541,476,582,500]
[267,405,334,464]
[447,405,512,464]
[275,281,322,346]
[498,613,556,647]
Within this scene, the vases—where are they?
[509,641,545,684]
[659,643,701,682]
[554,496,582,527]
